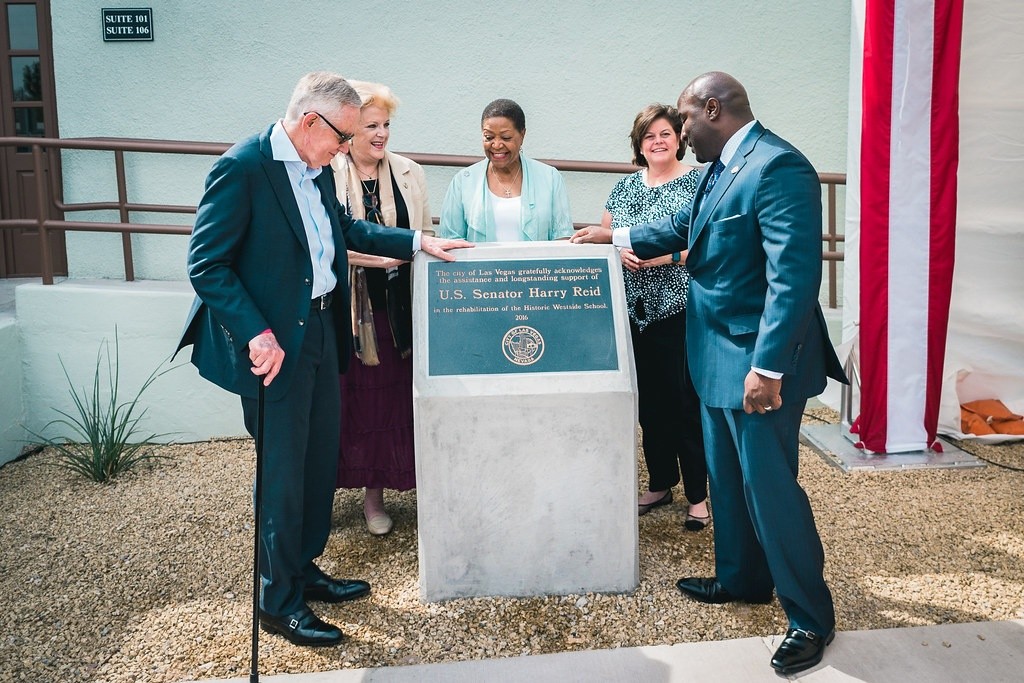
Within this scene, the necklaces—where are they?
[356,165,377,179]
[491,162,521,198]
[354,170,378,194]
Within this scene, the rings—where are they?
[764,406,772,411]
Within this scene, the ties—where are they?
[699,158,726,212]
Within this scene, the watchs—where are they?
[670,252,681,264]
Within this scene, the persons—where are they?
[568,71,851,674]
[440,96,574,244]
[596,102,711,532]
[170,70,474,647]
[323,77,436,535]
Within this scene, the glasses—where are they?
[362,192,383,226]
[304,111,354,145]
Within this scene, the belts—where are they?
[311,291,336,310]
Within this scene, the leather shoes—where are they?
[258,600,343,646]
[675,576,773,604]
[303,573,372,602]
[770,626,835,673]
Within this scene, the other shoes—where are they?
[685,496,710,532]
[637,488,674,516]
[362,500,393,535]
